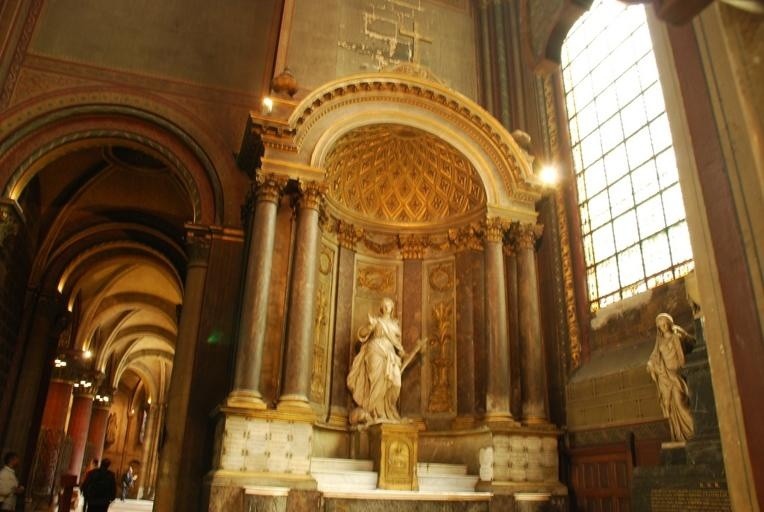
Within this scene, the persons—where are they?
[343,295,405,424]
[0,450,26,511]
[119,463,138,502]
[77,458,98,511]
[643,309,696,443]
[79,457,117,512]
